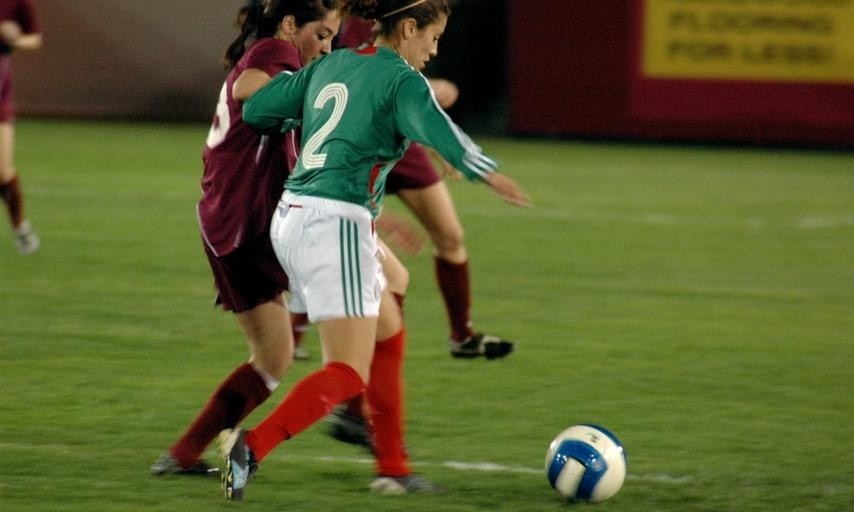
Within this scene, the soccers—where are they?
[545,425,627,502]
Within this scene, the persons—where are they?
[0,0,44,255]
[151,0,533,501]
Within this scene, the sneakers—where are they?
[371,474,443,498]
[15,221,39,256]
[327,403,369,446]
[450,334,514,360]
[218,428,258,501]
[150,453,217,478]
